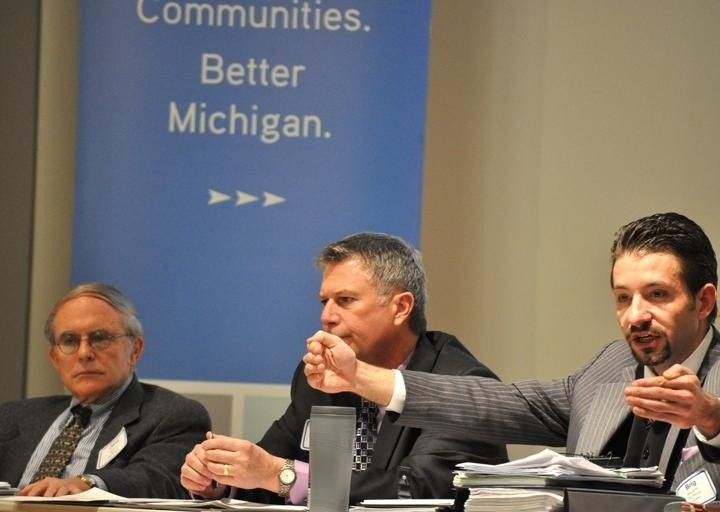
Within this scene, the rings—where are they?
[223,464,229,476]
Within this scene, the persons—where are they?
[303,213,720,502]
[0,283,212,498]
[180,231,511,512]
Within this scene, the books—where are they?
[452,448,686,512]
[346,498,457,512]
[0,481,18,497]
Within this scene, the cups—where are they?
[308,405,357,512]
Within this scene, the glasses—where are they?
[55,329,128,354]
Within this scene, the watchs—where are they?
[76,474,96,488]
[278,458,297,498]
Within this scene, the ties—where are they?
[28,403,92,484]
[351,396,379,472]
